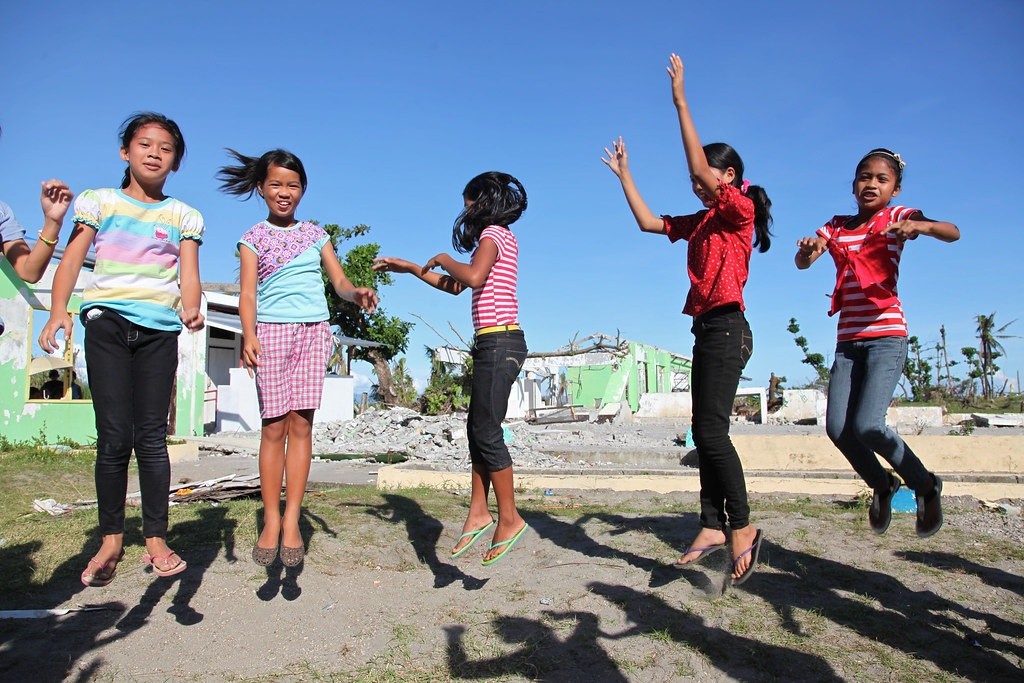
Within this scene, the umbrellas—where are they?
[29,355,73,376]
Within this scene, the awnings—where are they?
[207,309,385,347]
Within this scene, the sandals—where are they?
[917,473,943,538]
[868,472,901,533]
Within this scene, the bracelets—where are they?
[798,248,812,259]
[38,229,59,245]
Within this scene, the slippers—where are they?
[451,522,494,558]
[144,550,187,577]
[732,528,763,585]
[81,549,125,587]
[481,523,528,566]
[677,540,730,569]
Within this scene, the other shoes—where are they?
[252,527,280,565]
[280,516,305,567]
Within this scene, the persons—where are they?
[601,52,775,585]
[371,172,529,566]
[794,148,961,537]
[0,179,75,335]
[30,370,83,400]
[214,144,378,566]
[38,113,205,587]
